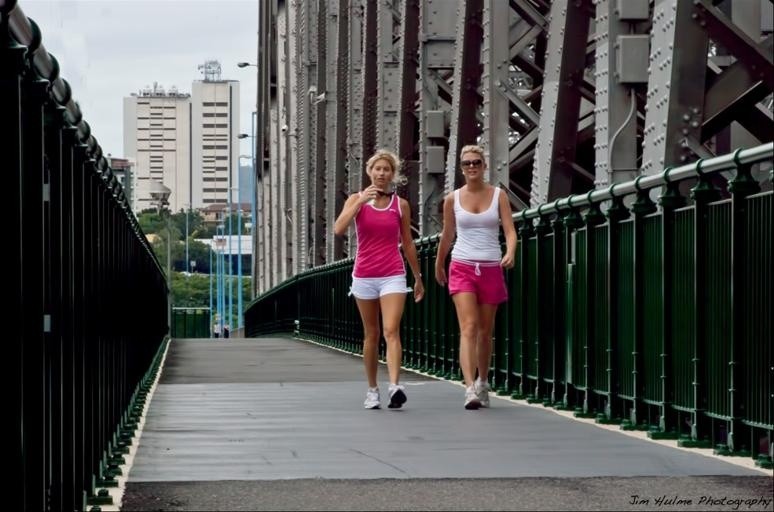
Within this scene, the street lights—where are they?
[207,152,258,337]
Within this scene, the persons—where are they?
[224,323,229,338]
[334,151,424,409]
[214,321,219,338]
[435,145,517,410]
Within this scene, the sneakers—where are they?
[387,384,407,408]
[464,377,489,408]
[365,387,380,409]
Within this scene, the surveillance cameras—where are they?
[280,124,289,134]
[305,85,317,96]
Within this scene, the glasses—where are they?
[376,183,395,197]
[462,160,481,165]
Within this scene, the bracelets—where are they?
[414,273,423,279]
